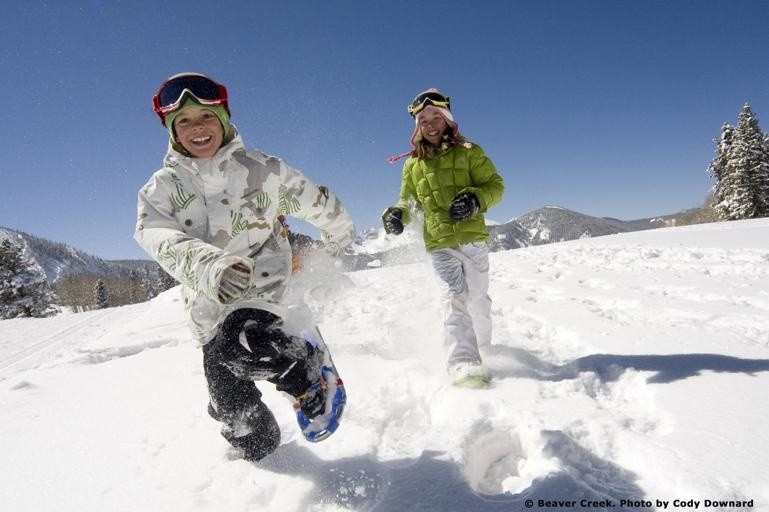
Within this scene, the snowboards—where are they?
[450,343,497,389]
[225,326,345,460]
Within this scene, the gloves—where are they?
[384,208,404,236]
[448,192,479,222]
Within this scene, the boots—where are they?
[299,346,328,419]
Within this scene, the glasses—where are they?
[407,92,450,120]
[152,74,229,122]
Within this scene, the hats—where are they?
[164,96,230,145]
[410,87,455,127]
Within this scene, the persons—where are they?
[381,88,505,387]
[133,71,357,463]
